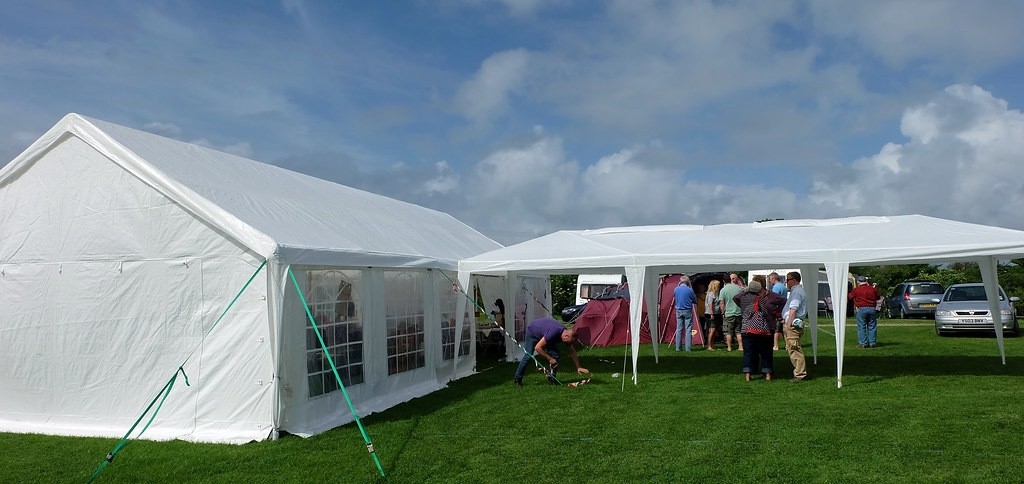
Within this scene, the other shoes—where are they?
[548,376,557,385]
[790,377,807,382]
[856,344,866,348]
[515,377,523,386]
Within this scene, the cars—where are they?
[930,283,1021,335]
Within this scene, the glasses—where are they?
[787,278,794,282]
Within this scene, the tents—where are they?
[568,271,744,345]
[1,111,511,446]
[449,208,1023,391]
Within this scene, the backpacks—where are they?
[746,295,771,335]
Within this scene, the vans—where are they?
[575,274,628,306]
[747,269,834,319]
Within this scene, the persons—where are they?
[491,299,505,329]
[739,279,775,381]
[730,275,788,375]
[514,315,590,387]
[847,273,884,348]
[671,270,790,353]
[780,270,810,383]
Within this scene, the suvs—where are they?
[886,279,945,319]
